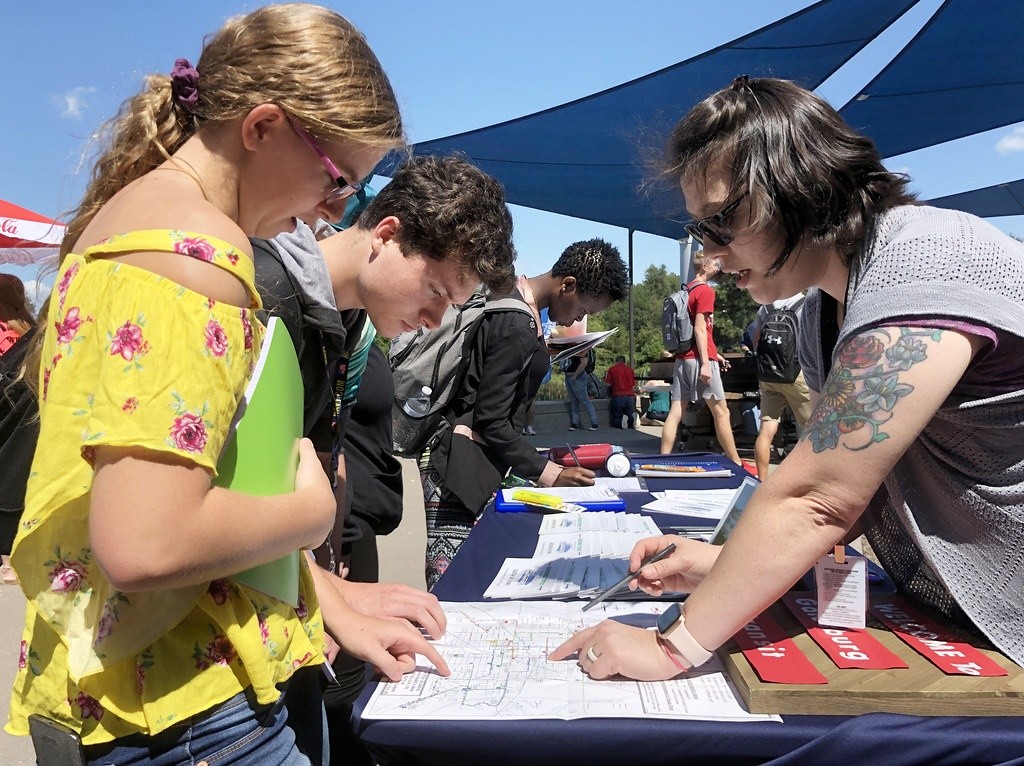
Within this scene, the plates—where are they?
[544,330,609,343]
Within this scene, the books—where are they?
[211,314,306,610]
[631,461,732,478]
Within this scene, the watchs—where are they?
[656,602,713,668]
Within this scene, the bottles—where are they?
[604,451,631,478]
[392,386,432,452]
[549,445,623,468]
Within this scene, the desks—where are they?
[350,451,1024,766]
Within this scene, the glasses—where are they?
[684,187,756,249]
[284,106,359,207]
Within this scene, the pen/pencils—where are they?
[659,526,715,539]
[582,543,677,612]
[641,464,706,472]
[566,442,581,467]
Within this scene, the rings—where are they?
[587,647,598,663]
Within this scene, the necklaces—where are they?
[172,155,214,204]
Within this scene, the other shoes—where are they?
[589,424,599,430]
[632,412,641,429]
[522,426,536,435]
[567,424,579,431]
[622,414,628,429]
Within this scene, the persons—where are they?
[0,273,50,585]
[565,357,601,430]
[546,78,1024,682]
[13,3,450,766]
[605,355,641,431]
[251,152,629,766]
[662,251,811,484]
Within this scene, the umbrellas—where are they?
[0,199,70,267]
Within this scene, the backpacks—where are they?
[661,280,706,355]
[585,347,596,373]
[386,280,537,457]
[757,297,806,383]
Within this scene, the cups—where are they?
[550,326,567,337]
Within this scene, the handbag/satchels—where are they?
[587,373,606,398]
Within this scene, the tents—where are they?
[380,0,1024,252]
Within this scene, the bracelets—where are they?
[655,629,688,674]
[572,374,578,379]
